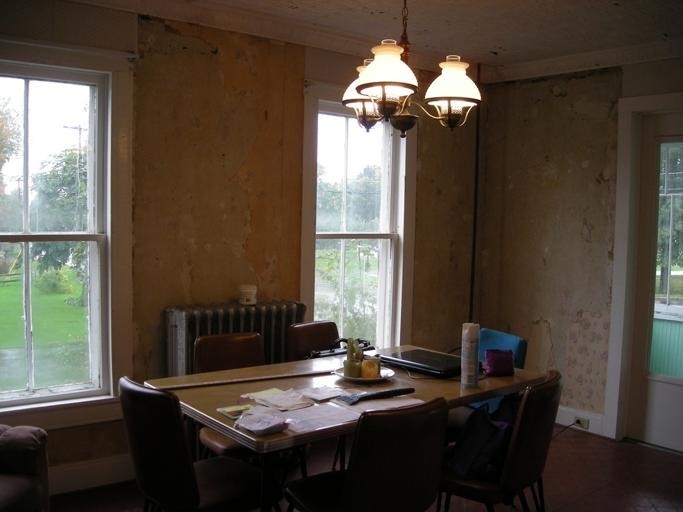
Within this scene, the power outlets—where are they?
[574,416,589,429]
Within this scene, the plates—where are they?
[330,366,396,383]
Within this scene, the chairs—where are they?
[284,397,448,512]
[434,370,563,512]
[119,375,276,512]
[450,329,527,444]
[290,321,348,471]
[192,332,290,466]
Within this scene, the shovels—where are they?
[337,388,415,406]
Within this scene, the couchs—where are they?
[0,423,49,512]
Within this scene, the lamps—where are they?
[341,1,482,139]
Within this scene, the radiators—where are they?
[166,297,307,461]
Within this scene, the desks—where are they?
[141,343,546,511]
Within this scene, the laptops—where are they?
[378,348,482,378]
